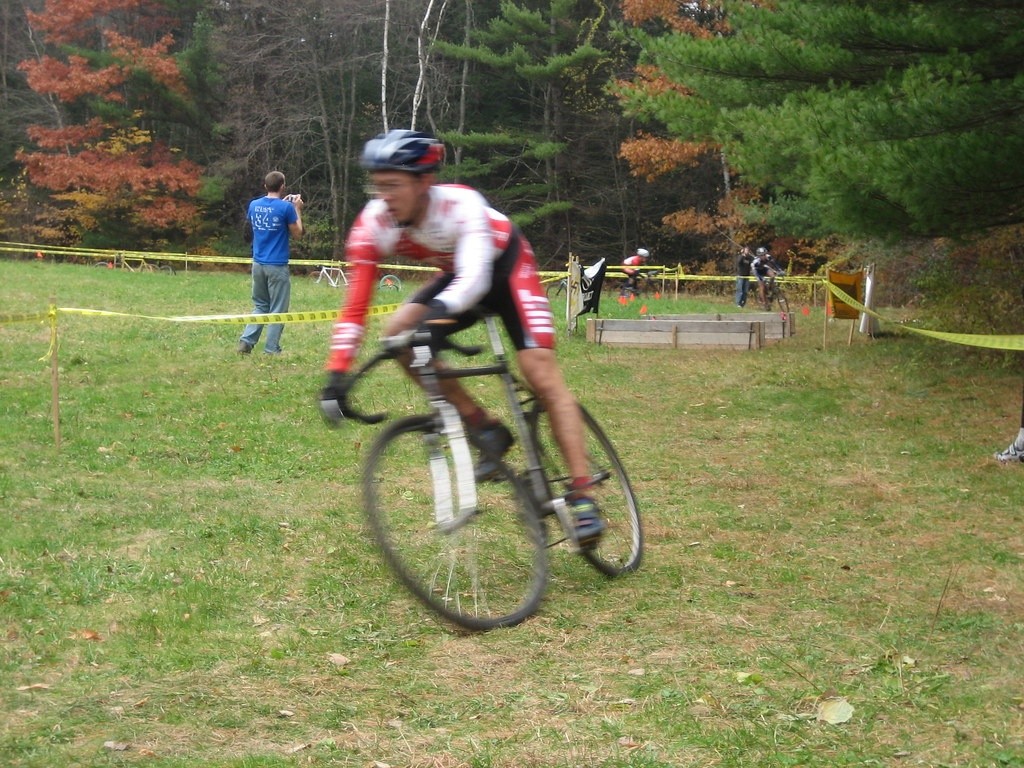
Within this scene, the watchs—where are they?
[424,298,447,318]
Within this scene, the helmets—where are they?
[636,248,650,258]
[757,247,767,256]
[363,129,446,172]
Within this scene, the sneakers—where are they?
[571,493,603,544]
[995,443,1024,463]
[469,421,513,477]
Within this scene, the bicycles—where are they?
[546,275,569,303]
[306,259,402,296]
[335,327,645,632]
[617,275,670,303]
[754,273,790,314]
[94,251,175,278]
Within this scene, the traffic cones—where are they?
[801,308,809,316]
[630,293,634,301]
[619,294,626,305]
[655,290,661,300]
[639,306,646,315]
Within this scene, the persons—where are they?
[734,246,786,308]
[235,172,304,359]
[621,249,649,296]
[993,402,1024,464]
[318,128,604,546]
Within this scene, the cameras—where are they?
[289,195,296,201]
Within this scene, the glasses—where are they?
[362,177,416,195]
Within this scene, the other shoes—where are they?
[737,304,741,308]
[239,341,252,354]
[765,303,771,312]
